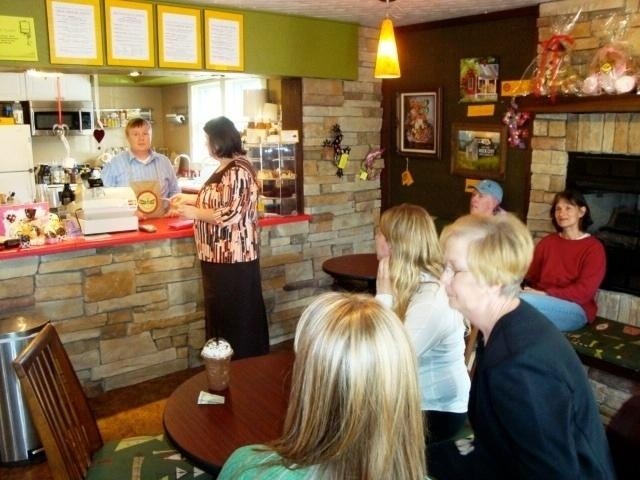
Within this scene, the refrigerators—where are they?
[0,125,37,204]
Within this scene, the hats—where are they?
[466,179,504,203]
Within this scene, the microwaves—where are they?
[29,101,95,137]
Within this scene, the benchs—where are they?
[562,316,640,383]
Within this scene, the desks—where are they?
[163,351,296,476]
[323,253,380,296]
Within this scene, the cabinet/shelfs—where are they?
[242,143,297,217]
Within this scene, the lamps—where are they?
[374,0,401,79]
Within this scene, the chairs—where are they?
[13,323,217,480]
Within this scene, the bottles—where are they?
[62,183,75,205]
[0,239,20,250]
[38,162,103,186]
[0,101,24,125]
[257,199,264,219]
[100,110,127,127]
[74,182,86,200]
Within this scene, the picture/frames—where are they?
[395,88,443,160]
[45,0,244,72]
[448,122,508,182]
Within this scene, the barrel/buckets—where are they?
[173,153,193,179]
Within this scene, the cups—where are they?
[247,122,281,144]
[36,183,61,211]
[202,339,234,391]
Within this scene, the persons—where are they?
[438,211,616,480]
[518,189,606,334]
[216,287,434,480]
[372,202,471,443]
[169,115,270,360]
[100,117,182,217]
[467,179,505,214]
